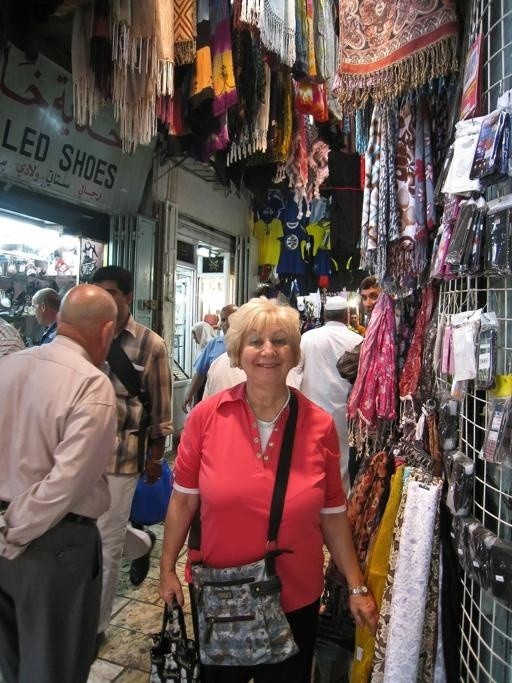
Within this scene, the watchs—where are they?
[347,585,371,596]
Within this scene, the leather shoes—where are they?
[129,528,156,587]
[90,631,104,664]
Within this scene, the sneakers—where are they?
[0,254,77,317]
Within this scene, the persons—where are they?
[181,304,240,414]
[2,319,25,357]
[156,295,383,683]
[72,266,174,662]
[32,287,61,345]
[202,352,247,400]
[285,295,364,497]
[359,274,383,319]
[2,279,121,683]
[350,313,365,337]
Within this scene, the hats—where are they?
[325,296,348,311]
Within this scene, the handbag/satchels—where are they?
[132,460,174,525]
[148,594,200,683]
[187,557,302,667]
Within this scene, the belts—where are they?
[0,498,97,530]
